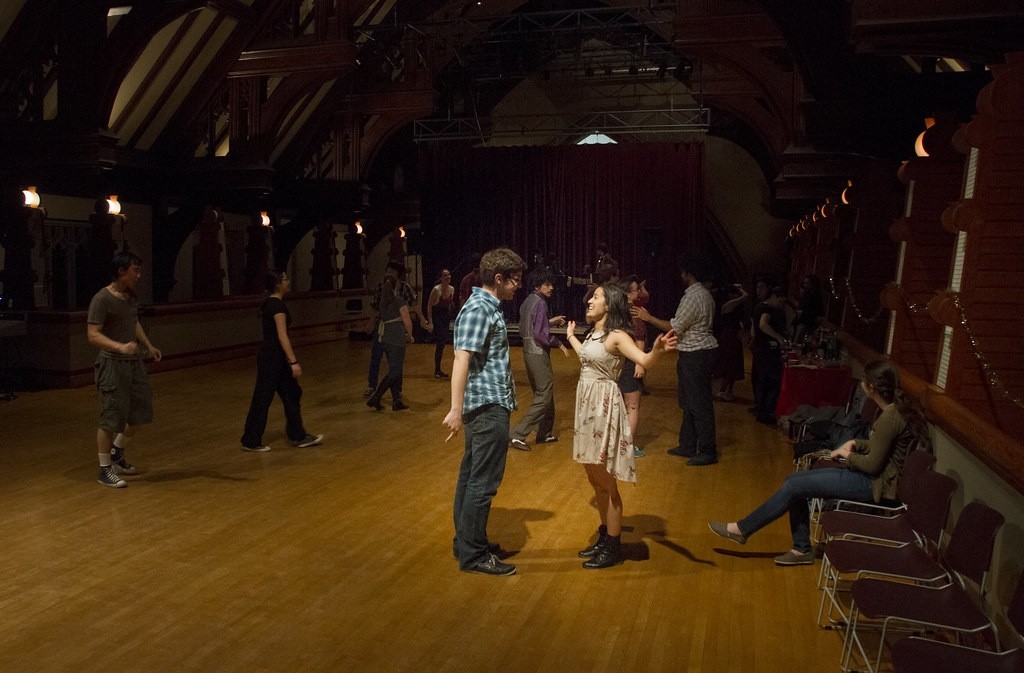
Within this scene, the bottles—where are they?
[764,340,789,350]
[801,329,837,360]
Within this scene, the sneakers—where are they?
[453,544,500,559]
[298,434,324,447]
[242,444,270,451]
[98,464,127,487]
[463,553,516,575]
[110,442,136,474]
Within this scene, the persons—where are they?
[702,271,747,401]
[427,269,455,379]
[706,359,928,566]
[566,283,678,570]
[582,250,649,467]
[361,259,426,411]
[458,267,481,305]
[752,274,824,426]
[239,268,325,452]
[629,260,720,466]
[441,248,529,578]
[508,268,570,451]
[86,251,162,488]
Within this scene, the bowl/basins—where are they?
[788,360,799,365]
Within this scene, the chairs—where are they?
[788,385,1024,673]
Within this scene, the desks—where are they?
[773,340,859,417]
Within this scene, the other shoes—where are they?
[774,550,814,564]
[508,437,531,450]
[536,435,558,443]
[392,400,409,410]
[686,456,718,465]
[708,521,746,544]
[668,446,696,457]
[435,371,448,378]
[367,397,385,410]
[634,447,645,457]
[717,392,736,401]
[362,387,376,396]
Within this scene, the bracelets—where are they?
[287,359,301,366]
[567,335,574,341]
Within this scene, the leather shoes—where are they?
[583,547,624,568]
[578,541,606,558]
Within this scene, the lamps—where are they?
[103,193,122,215]
[575,129,618,145]
[23,185,42,208]
[398,226,406,238]
[355,219,363,234]
[260,208,271,226]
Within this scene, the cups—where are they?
[788,352,796,359]
